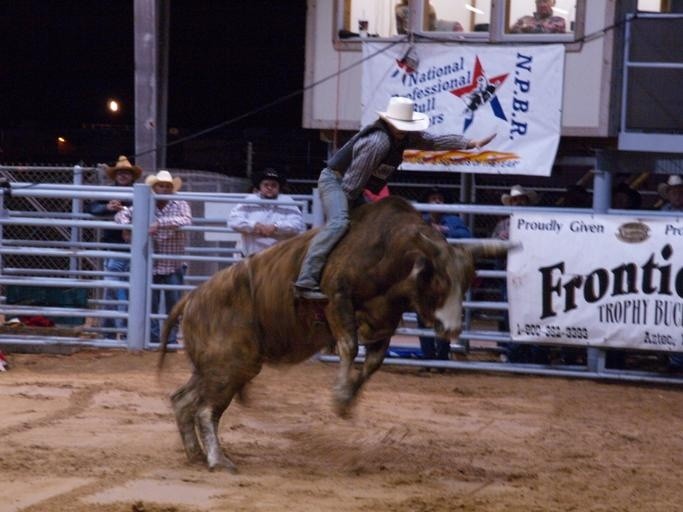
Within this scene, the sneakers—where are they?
[294,288,328,302]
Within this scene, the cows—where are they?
[154,193,525,476]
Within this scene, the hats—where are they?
[501,184,540,208]
[104,155,143,181]
[253,167,287,189]
[657,174,682,200]
[375,95,429,133]
[144,169,182,193]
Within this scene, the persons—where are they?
[508,1,566,35]
[113,170,191,347]
[294,94,432,305]
[89,157,143,341]
[223,161,300,261]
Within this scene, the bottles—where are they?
[357,9,369,40]
